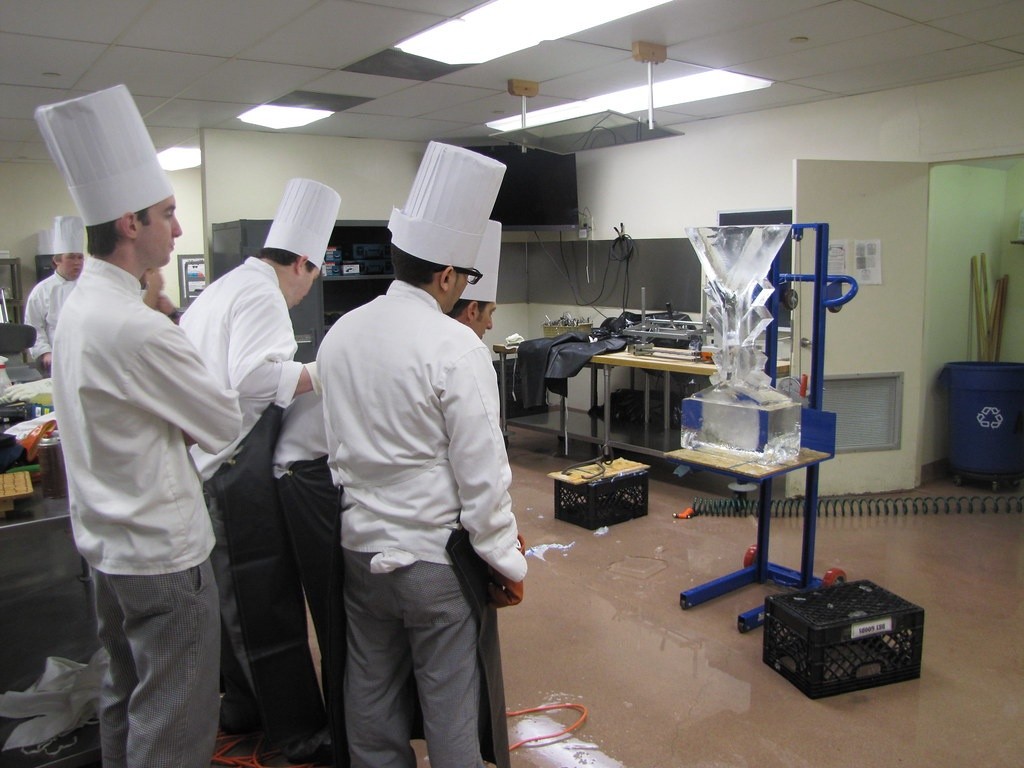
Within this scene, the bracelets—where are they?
[168,311,180,321]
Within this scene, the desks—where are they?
[494,344,790,500]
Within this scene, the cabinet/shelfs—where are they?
[211,219,395,366]
[0,257,28,363]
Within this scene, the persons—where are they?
[24,243,84,369]
[52,177,528,768]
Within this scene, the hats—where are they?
[388,141,506,268]
[52,215,84,254]
[263,177,342,271]
[34,83,174,226]
[458,220,502,302]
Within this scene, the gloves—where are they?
[487,533,525,610]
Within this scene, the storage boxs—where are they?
[554,471,649,530]
[762,579,925,700]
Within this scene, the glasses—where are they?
[431,268,481,285]
